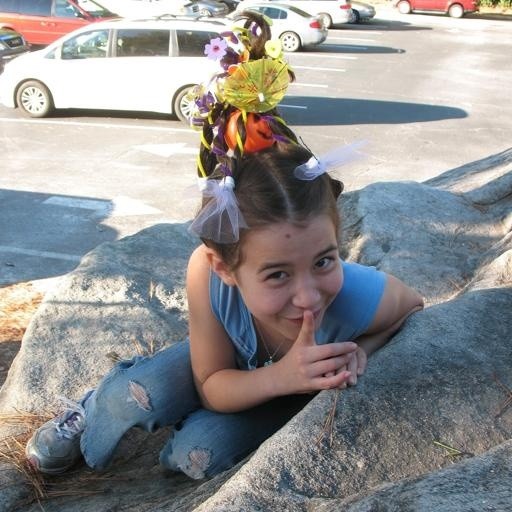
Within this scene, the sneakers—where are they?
[25,389,96,474]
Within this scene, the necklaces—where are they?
[253,320,287,367]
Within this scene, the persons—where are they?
[25,10,425,487]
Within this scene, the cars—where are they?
[1,15,246,126]
[133,0,376,52]
[389,0,481,19]
[0,1,125,54]
